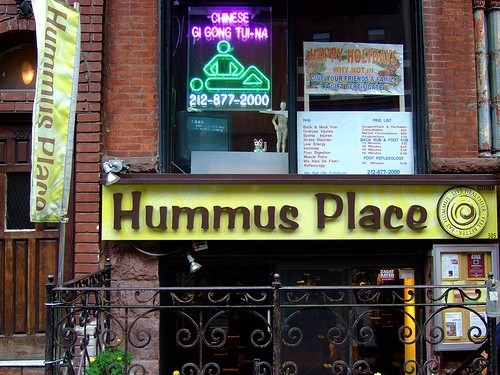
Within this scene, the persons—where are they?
[271,102,289,152]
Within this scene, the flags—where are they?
[31,0,80,224]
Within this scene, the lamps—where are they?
[102,159,128,186]
[186,251,202,273]
